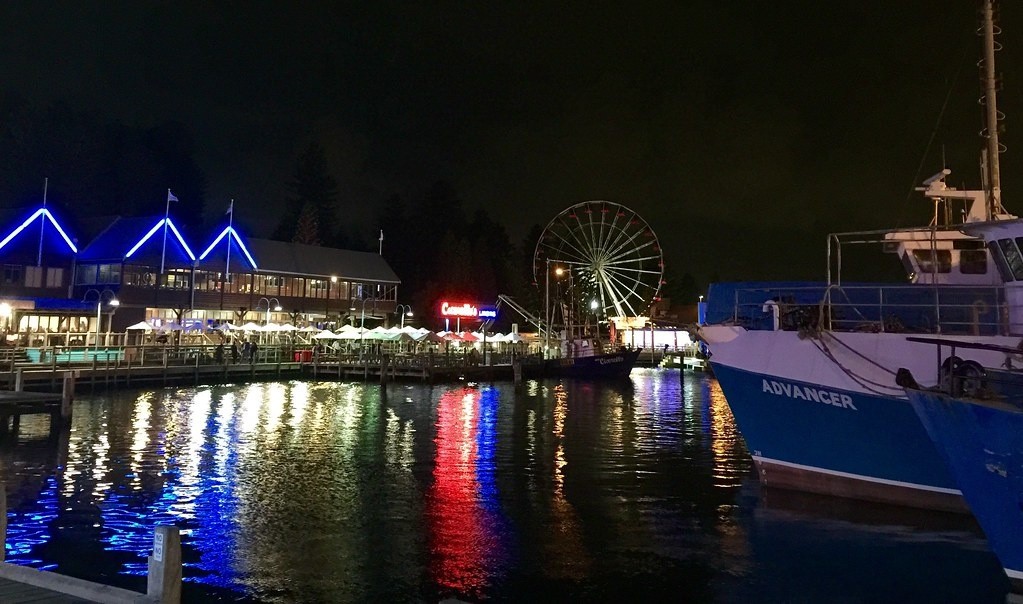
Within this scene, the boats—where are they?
[545,336,642,380]
[520,356,552,381]
[895,339,1023,592]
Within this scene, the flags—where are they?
[169,192,178,201]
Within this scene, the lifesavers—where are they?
[942,355,964,385]
[952,360,989,398]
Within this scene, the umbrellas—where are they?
[126,321,159,344]
[313,329,337,339]
[437,330,522,345]
[335,324,356,332]
[279,324,300,344]
[187,323,211,343]
[160,322,186,345]
[359,326,447,342]
[239,322,261,334]
[214,321,242,343]
[259,323,280,345]
[299,325,322,343]
[336,330,361,340]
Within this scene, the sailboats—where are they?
[686,0,1022,514]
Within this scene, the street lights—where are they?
[256,297,282,364]
[350,298,377,365]
[82,289,119,353]
[394,304,414,350]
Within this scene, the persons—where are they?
[215,341,258,365]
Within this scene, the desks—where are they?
[319,353,462,366]
